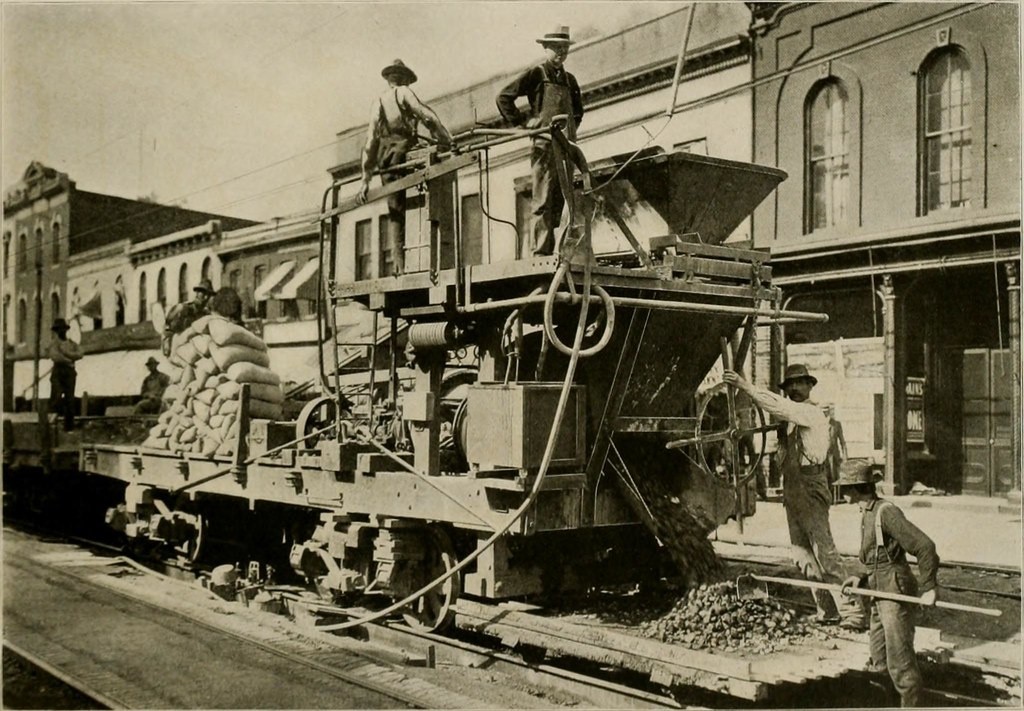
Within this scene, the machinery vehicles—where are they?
[3,144,830,635]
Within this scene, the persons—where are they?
[133,357,170,415]
[46,317,83,415]
[831,460,941,710]
[493,26,586,257]
[721,363,850,586]
[354,57,454,276]
[162,279,219,358]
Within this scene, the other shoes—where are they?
[808,613,841,624]
[840,613,869,628]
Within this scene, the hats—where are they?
[51,318,70,330]
[822,406,830,411]
[382,60,418,84]
[832,459,883,485]
[194,279,217,296]
[777,364,818,390]
[145,357,160,364]
[536,25,576,45]
